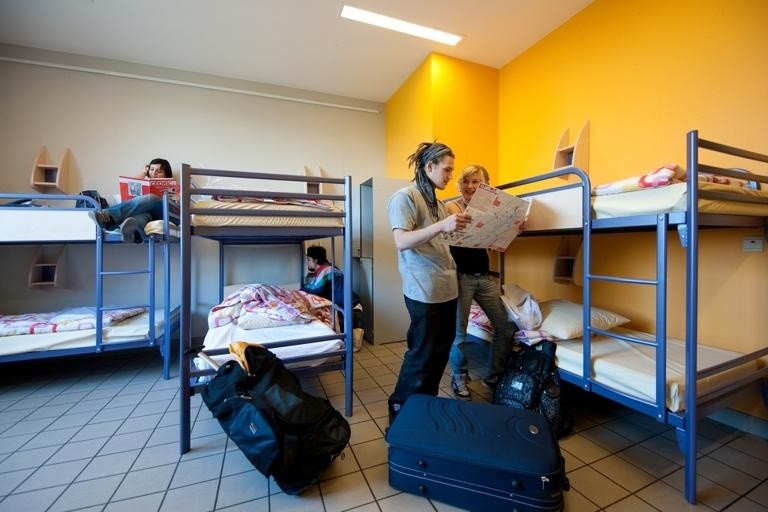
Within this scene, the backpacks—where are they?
[492,340,572,440]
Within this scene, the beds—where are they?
[443,130,766,508]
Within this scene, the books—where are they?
[118,175,181,202]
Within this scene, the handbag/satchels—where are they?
[76,191,108,211]
[201,346,351,496]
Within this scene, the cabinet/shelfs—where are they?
[351,172,413,345]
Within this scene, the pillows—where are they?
[539,298,632,341]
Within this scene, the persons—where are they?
[382,137,471,445]
[445,165,531,398]
[87,158,195,248]
[299,244,363,354]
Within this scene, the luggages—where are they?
[385,393,570,511]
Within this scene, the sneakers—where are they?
[87,209,114,231]
[451,373,474,402]
[121,216,138,245]
[352,328,365,352]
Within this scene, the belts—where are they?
[473,270,500,278]
